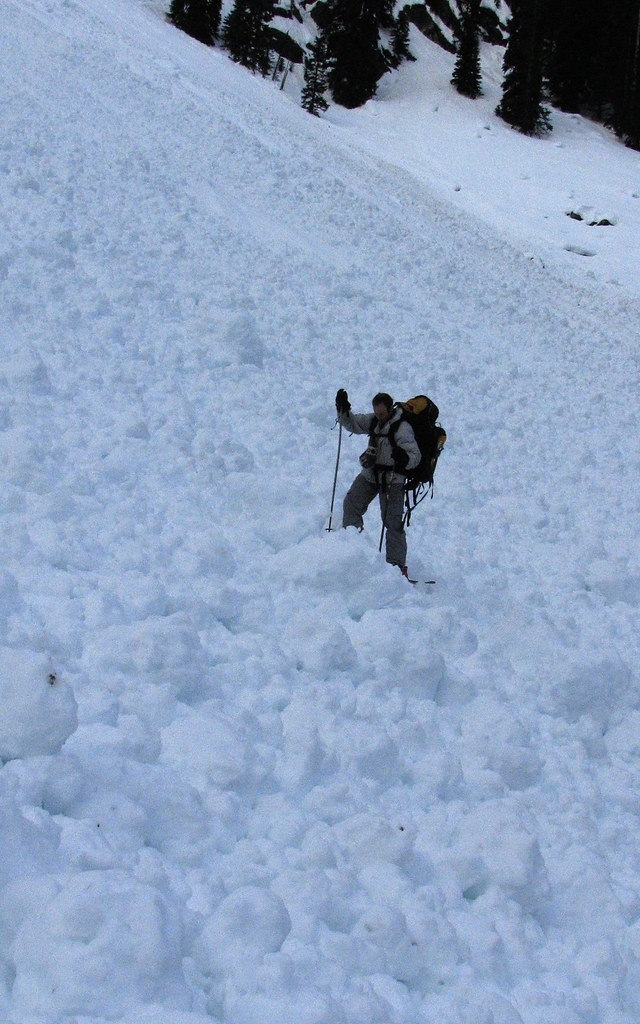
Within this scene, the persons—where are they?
[336,387,423,583]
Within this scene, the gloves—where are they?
[391,447,409,466]
[336,389,351,411]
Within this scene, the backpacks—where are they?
[368,395,447,483]
